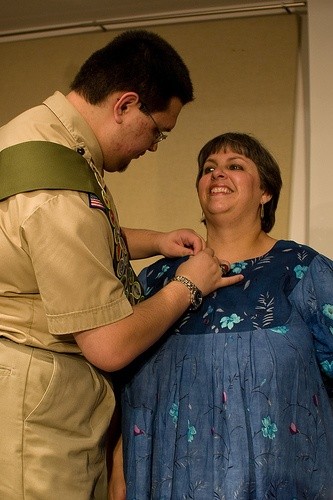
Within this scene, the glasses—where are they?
[139,101,168,144]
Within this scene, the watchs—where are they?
[165,276,202,312]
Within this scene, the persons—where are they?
[0,29,246,500]
[117,132,333,500]
